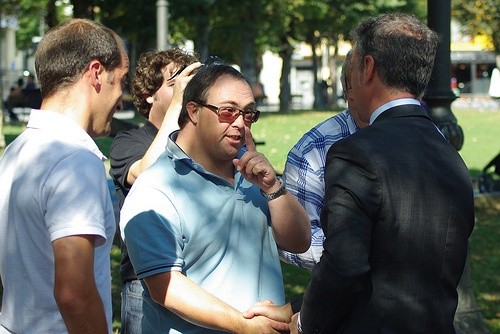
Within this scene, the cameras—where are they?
[188,56,225,76]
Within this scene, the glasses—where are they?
[196,101,260,124]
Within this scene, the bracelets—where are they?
[297,322,303,334]
[260,176,286,200]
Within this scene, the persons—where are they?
[6,74,37,122]
[0,19,130,334]
[119,66,312,334]
[483,152,500,191]
[108,47,240,334]
[276,50,370,271]
[242,13,476,334]
